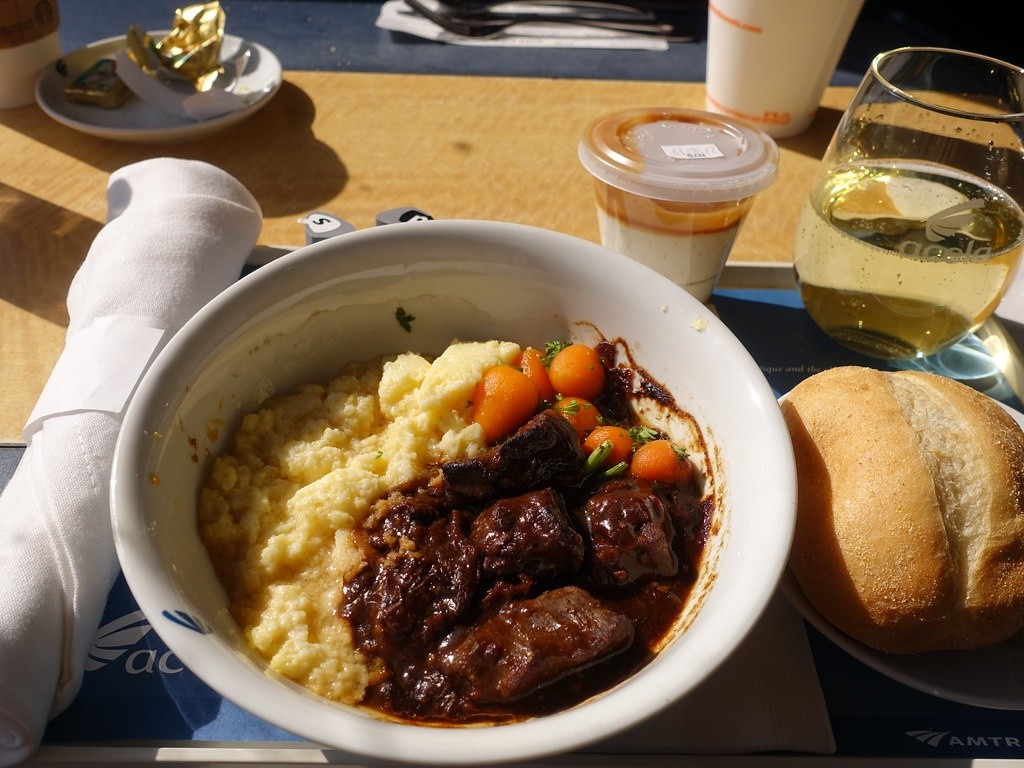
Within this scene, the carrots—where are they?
[470,342,695,483]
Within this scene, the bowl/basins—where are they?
[110,221,796,765]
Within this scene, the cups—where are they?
[0,0,60,109]
[705,0,864,138]
[794,47,1024,361]
[578,108,779,306]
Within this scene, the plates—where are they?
[34,30,282,141]
[778,389,1024,709]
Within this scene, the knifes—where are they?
[397,11,653,21]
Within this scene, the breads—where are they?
[773,366,1024,655]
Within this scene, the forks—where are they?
[404,0,697,41]
